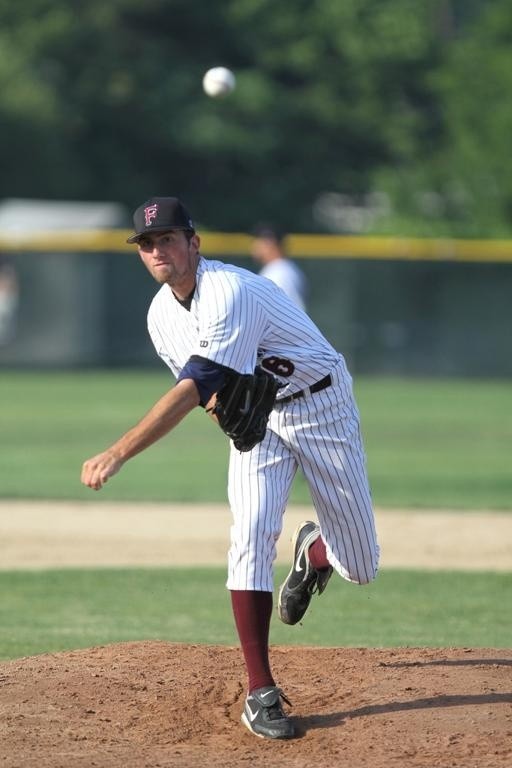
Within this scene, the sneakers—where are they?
[242,687,294,739]
[277,521,333,625]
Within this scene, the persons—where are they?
[246,222,309,312]
[82,196,380,740]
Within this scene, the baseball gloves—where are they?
[216,369,278,453]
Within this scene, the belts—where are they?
[276,374,331,403]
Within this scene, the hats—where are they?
[126,198,194,243]
